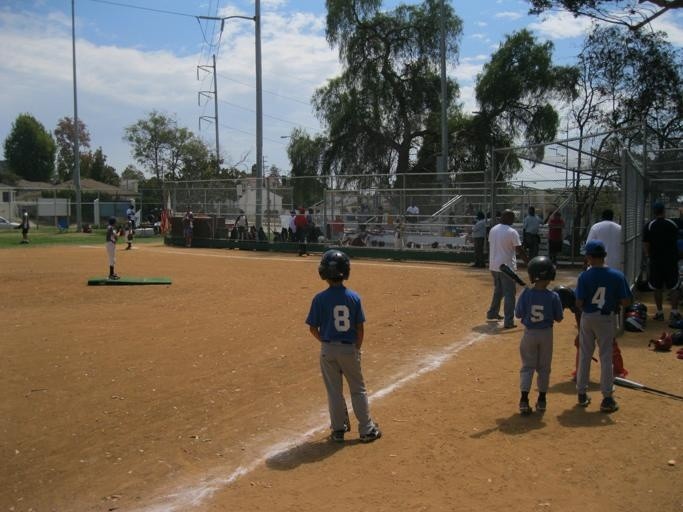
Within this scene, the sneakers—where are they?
[504,325,517,330]
[360,428,381,442]
[520,400,529,410]
[578,398,590,406]
[601,401,620,411]
[109,274,121,280]
[536,400,547,409]
[487,314,504,321]
[332,431,344,442]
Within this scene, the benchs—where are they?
[508,221,550,254]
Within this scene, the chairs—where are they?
[228,224,363,247]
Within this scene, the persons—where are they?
[106,205,136,279]
[234,199,419,247]
[182,207,194,247]
[304,250,382,442]
[16,209,30,244]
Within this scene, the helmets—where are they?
[319,250,350,280]
[649,330,673,352]
[528,255,556,283]
[550,286,576,309]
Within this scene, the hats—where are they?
[581,239,608,256]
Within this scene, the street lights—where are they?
[281,135,296,142]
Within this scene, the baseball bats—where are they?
[614,375,682,401]
[500,264,529,289]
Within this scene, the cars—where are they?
[0,216,21,228]
